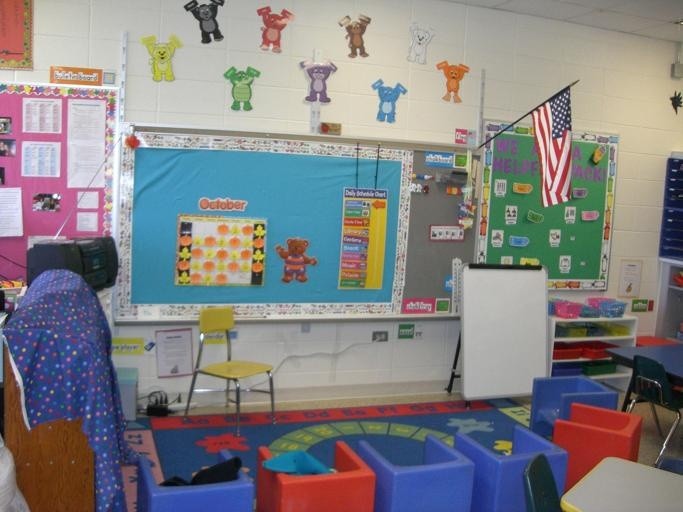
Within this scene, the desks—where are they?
[602,344,682,412]
[561,456,682,512]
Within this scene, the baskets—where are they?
[548,298,630,376]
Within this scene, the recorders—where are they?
[25,236,117,291]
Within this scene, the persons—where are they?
[35,196,56,211]
[0,141,6,156]
[3,144,14,156]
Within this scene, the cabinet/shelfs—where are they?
[547,315,638,392]
[652,157,681,343]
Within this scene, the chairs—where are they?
[623,355,682,467]
[183,306,276,438]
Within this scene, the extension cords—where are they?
[142,399,197,412]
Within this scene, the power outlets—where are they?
[371,331,388,343]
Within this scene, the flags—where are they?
[530,88,572,208]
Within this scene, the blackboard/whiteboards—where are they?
[110,123,476,327]
